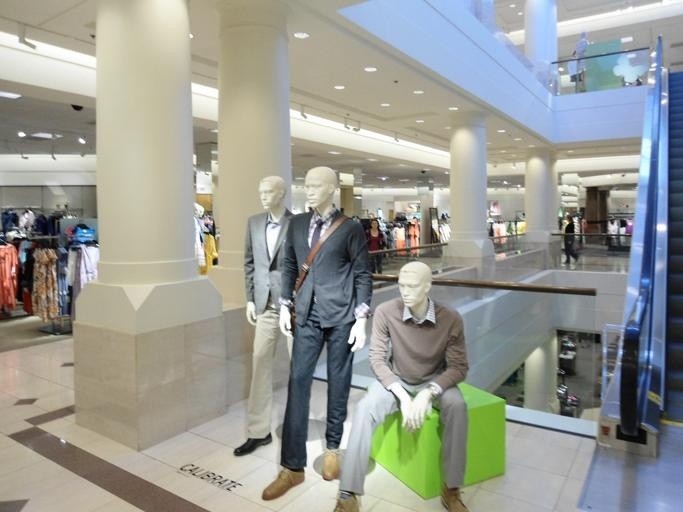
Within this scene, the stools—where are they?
[369,380,507,498]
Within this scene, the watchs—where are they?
[428,384,444,400]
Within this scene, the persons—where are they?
[234,174,293,456]
[562,213,581,267]
[332,259,469,512]
[262,166,372,500]
[366,219,385,275]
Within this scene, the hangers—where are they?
[27,234,98,258]
[0,206,55,216]
[0,229,42,249]
[52,207,83,219]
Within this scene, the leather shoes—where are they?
[262,467,305,501]
[335,491,359,511]
[321,451,340,483]
[234,432,274,458]
[441,487,470,512]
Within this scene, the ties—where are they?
[310,211,337,252]
